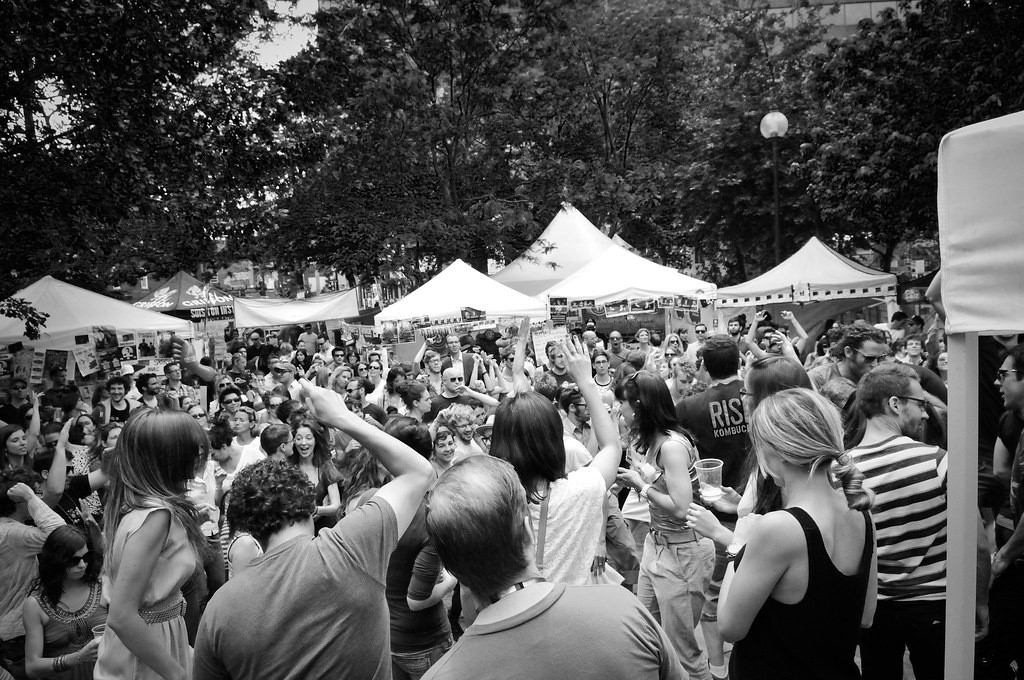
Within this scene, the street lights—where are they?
[760,111,789,267]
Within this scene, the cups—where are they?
[91,623,106,638]
[694,459,724,503]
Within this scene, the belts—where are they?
[649,529,703,548]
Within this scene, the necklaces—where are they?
[476,578,547,615]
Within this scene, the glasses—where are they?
[764,335,770,340]
[609,337,620,341]
[594,360,607,364]
[996,368,1018,380]
[628,370,648,387]
[576,403,588,410]
[854,349,888,363]
[65,466,76,474]
[664,330,705,361]
[908,323,919,327]
[586,327,595,331]
[896,395,930,411]
[737,388,756,404]
[68,550,92,568]
[822,344,828,348]
[12,384,28,391]
[169,326,518,444]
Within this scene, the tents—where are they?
[374,259,549,333]
[488,200,716,295]
[936,110,1024,680]
[717,236,898,328]
[532,244,717,302]
[0,275,195,349]
[131,270,239,332]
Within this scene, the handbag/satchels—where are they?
[83,499,102,547]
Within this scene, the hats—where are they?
[906,316,925,327]
[475,414,497,434]
[48,364,67,378]
[7,377,29,385]
[119,365,135,377]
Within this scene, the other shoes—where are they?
[447,609,465,642]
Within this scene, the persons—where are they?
[0,310,1024,680]
[193,378,437,679]
[22,525,109,680]
[615,369,716,680]
[716,388,879,680]
[417,454,690,680]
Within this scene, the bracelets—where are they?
[52,655,69,674]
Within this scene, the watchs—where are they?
[725,543,741,563]
[640,484,653,501]
